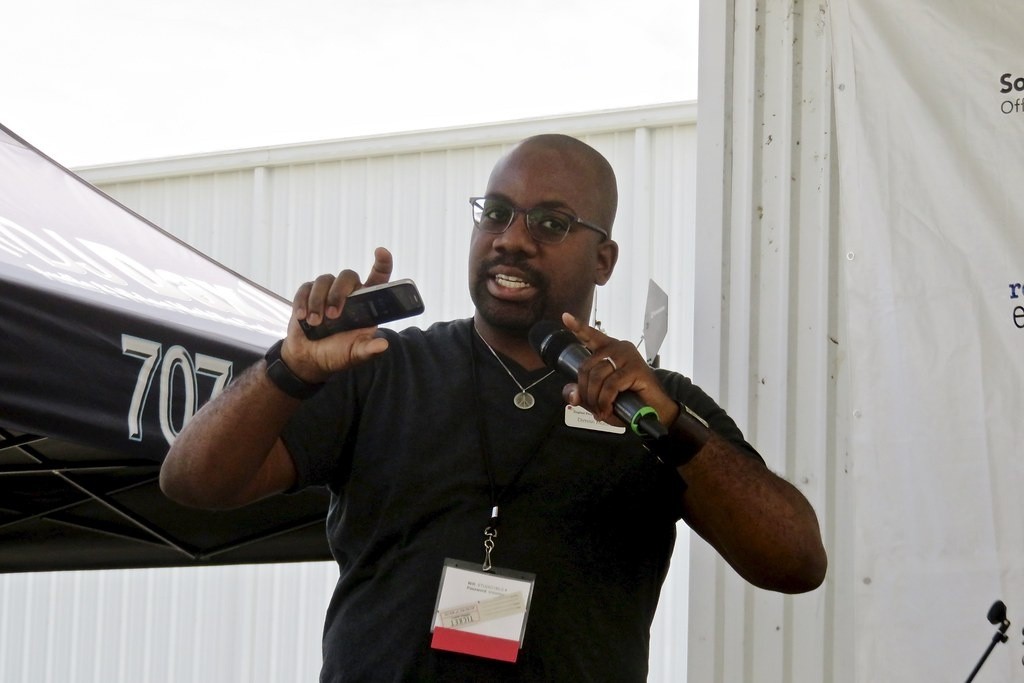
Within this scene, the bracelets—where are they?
[264,338,327,400]
[639,398,711,468]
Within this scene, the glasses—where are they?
[469,197,608,245]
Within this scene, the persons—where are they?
[158,133,827,683]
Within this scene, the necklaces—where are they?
[469,314,561,409]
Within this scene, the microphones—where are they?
[529,318,668,440]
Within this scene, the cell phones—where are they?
[298,279,425,341]
